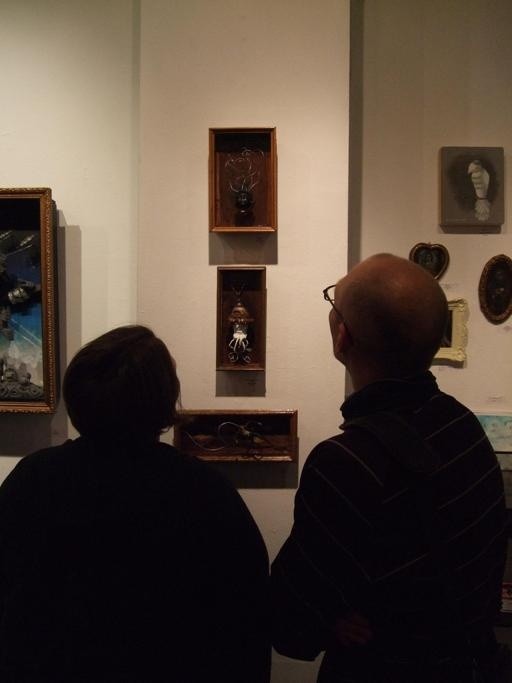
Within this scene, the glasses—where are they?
[323,282,353,341]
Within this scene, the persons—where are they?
[266,252,509,683]
[0,324,270,682]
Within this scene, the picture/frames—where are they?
[433,297,469,363]
[479,253,512,324]
[408,241,450,281]
[1,186,61,413]
